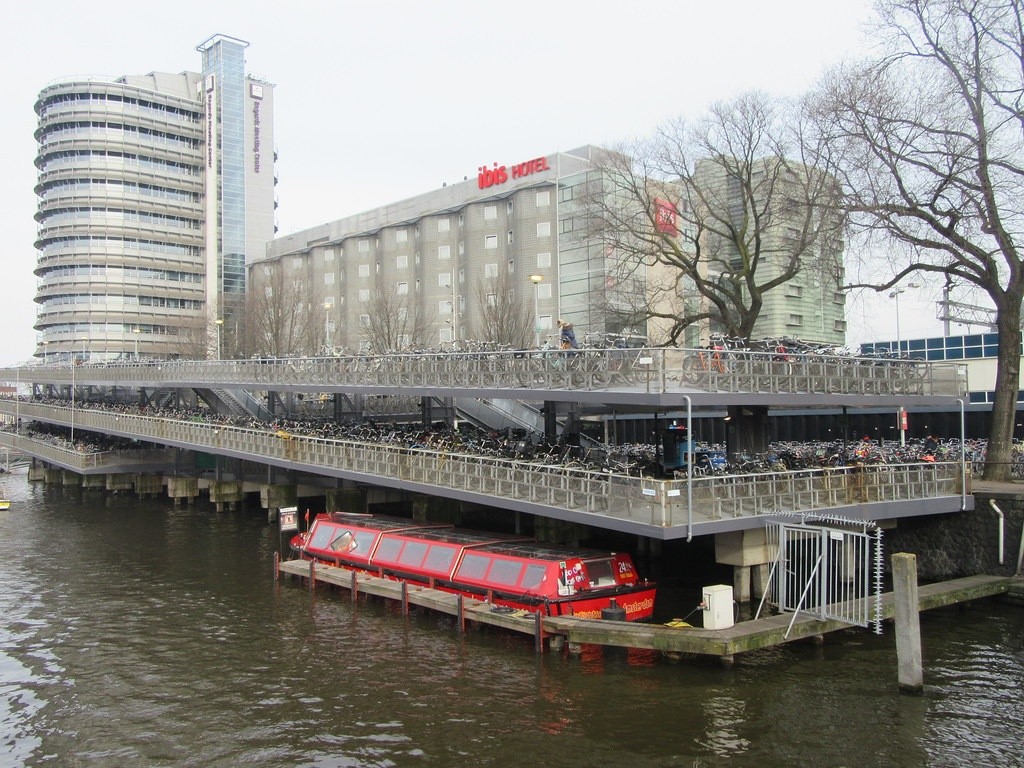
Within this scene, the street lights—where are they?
[132,329,140,358]
[529,275,542,347]
[43,342,49,367]
[215,319,224,359]
[81,336,87,361]
[889,285,905,359]
[323,304,330,347]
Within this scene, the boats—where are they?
[289,512,658,623]
[0,491,12,510]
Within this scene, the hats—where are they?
[556,320,565,327]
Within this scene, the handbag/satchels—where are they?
[921,455,934,461]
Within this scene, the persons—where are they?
[925,434,942,458]
[555,319,578,358]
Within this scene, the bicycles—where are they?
[1,328,1024,480]
